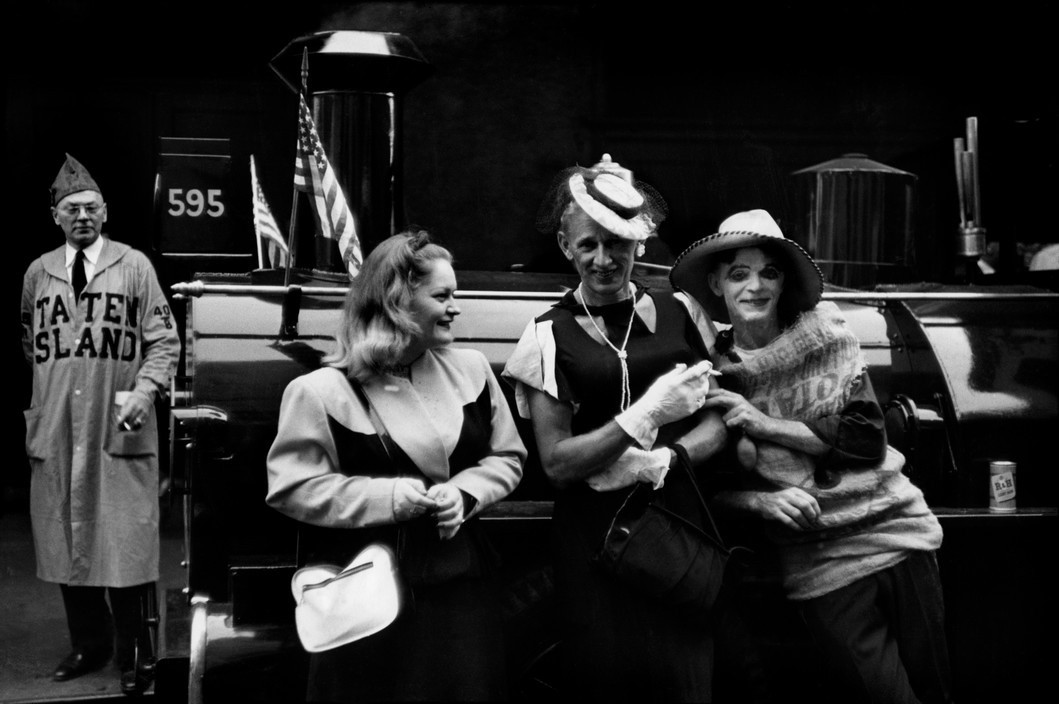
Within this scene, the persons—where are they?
[265,231,530,704]
[670,208,946,704]
[503,153,736,704]
[23,153,182,694]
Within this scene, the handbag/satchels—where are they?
[292,543,404,653]
[600,443,731,613]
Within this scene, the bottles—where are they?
[114,391,142,435]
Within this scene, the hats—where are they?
[669,210,824,324]
[50,152,101,207]
[568,154,667,240]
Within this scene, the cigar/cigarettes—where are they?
[117,415,131,431]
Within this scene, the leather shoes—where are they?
[122,670,152,692]
[53,647,100,681]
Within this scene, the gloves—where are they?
[586,445,671,492]
[614,360,713,451]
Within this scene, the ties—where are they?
[72,250,88,305]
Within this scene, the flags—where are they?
[293,93,364,284]
[252,159,293,270]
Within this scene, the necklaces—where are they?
[580,282,640,414]
